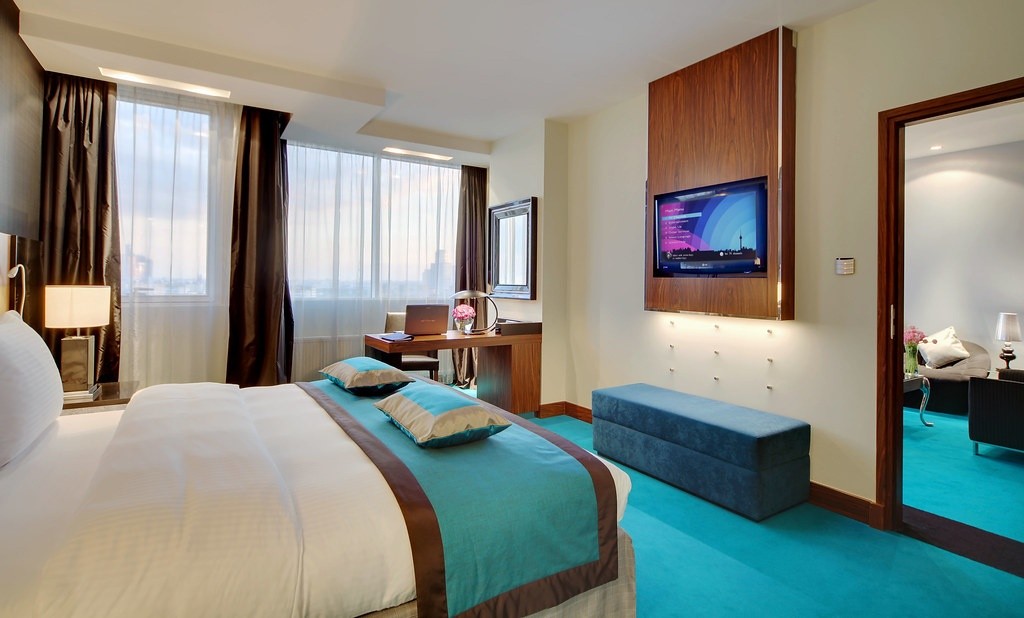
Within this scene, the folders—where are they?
[381,332,413,341]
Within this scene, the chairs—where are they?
[968,368,1024,456]
[385,312,439,382]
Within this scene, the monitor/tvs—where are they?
[654,176,768,279]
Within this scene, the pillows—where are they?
[318,356,415,398]
[0,310,64,468]
[373,384,512,449]
[919,326,970,369]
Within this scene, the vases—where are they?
[454,318,474,332]
[904,345,918,379]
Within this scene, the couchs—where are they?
[904,336,991,416]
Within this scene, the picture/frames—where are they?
[488,196,537,301]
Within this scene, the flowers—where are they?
[451,304,476,330]
[904,328,925,374]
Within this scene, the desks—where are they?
[365,329,542,414]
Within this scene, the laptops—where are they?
[394,305,450,335]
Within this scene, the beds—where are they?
[0,368,639,618]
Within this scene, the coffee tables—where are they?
[904,375,934,426]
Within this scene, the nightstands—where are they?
[62,380,139,410]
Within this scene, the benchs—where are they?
[592,382,811,523]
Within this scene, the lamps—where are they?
[994,313,1022,369]
[44,283,111,399]
[449,290,499,335]
